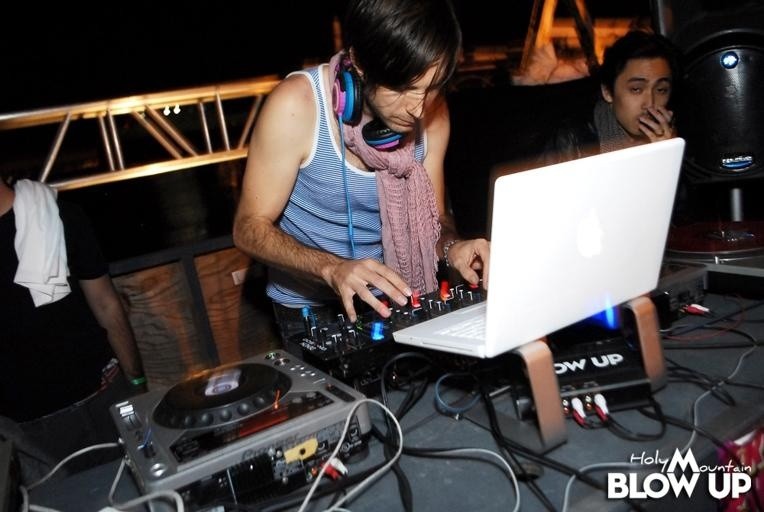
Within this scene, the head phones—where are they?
[332,50,404,152]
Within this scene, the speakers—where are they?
[674,26,764,188]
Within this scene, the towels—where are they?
[12,178,73,308]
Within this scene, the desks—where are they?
[21,288,763,512]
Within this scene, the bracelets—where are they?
[443,237,460,268]
[130,376,147,386]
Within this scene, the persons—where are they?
[0,178,148,487]
[229,0,492,347]
[533,29,677,166]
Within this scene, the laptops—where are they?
[392,136,687,362]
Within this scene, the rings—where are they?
[654,130,665,137]
